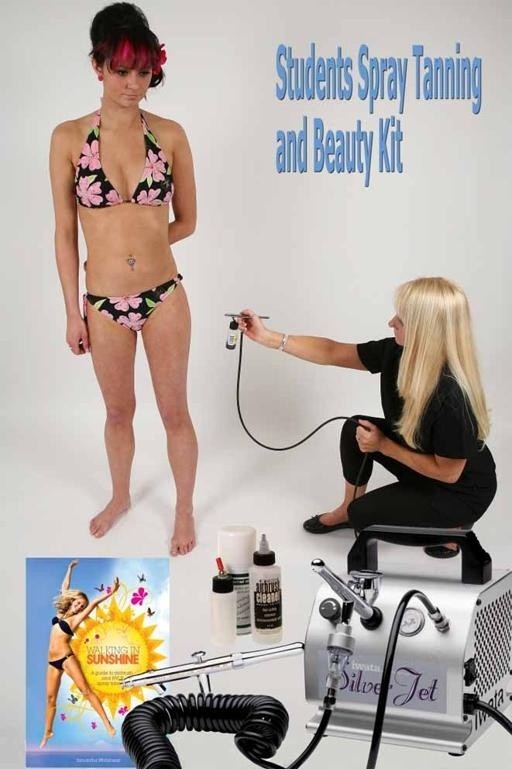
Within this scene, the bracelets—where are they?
[276,333,289,352]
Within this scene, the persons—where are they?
[49,2,198,556]
[39,559,119,749]
[238,276,497,559]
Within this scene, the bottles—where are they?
[209,558,238,649]
[217,524,257,636]
[247,534,284,646]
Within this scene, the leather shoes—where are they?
[425,545,459,558]
[303,514,354,533]
